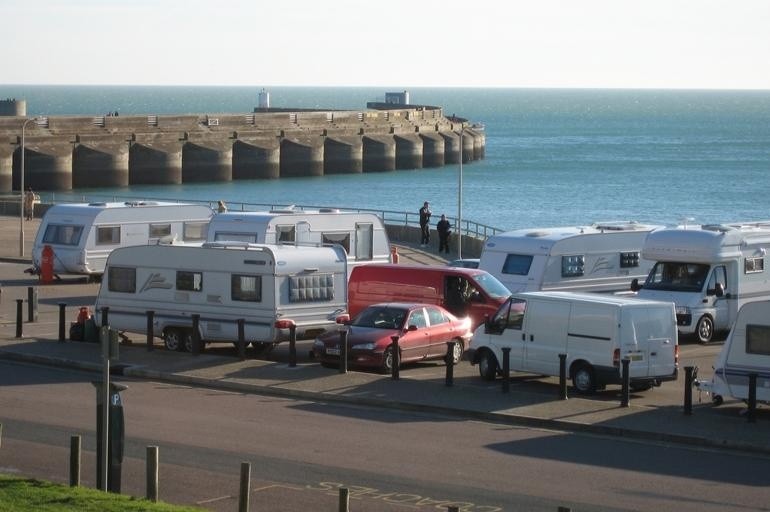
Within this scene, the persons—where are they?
[419,201,433,249]
[437,214,451,254]
[24,186,35,221]
[216,201,226,212]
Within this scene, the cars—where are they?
[311,301,473,373]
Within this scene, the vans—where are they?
[467,290,679,394]
[630,225,770,344]
[347,262,516,334]
